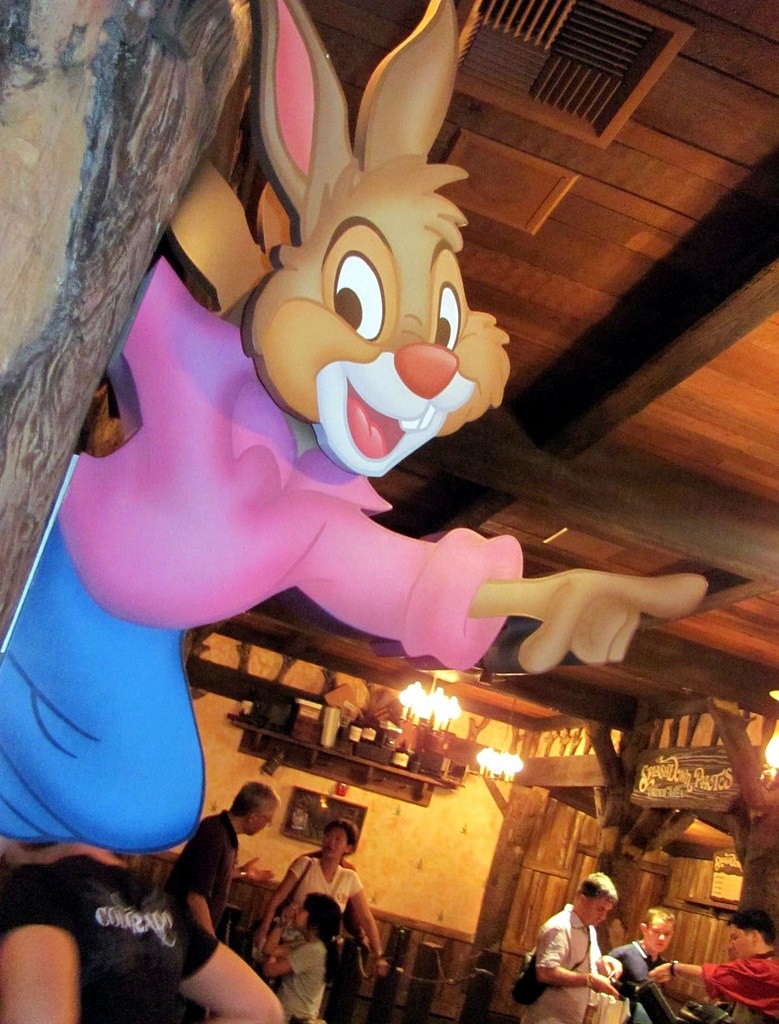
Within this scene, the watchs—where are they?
[670,960,679,976]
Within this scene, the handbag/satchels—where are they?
[253,898,288,946]
[514,954,548,1003]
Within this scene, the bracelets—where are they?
[586,972,593,988]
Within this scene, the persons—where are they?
[251,819,389,986]
[648,903,779,1024]
[517,873,624,1023]
[290,847,373,955]
[0,836,285,1023]
[162,780,282,1023]
[606,906,676,1024]
[263,891,345,1024]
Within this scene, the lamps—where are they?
[473,698,530,784]
[397,668,467,731]
